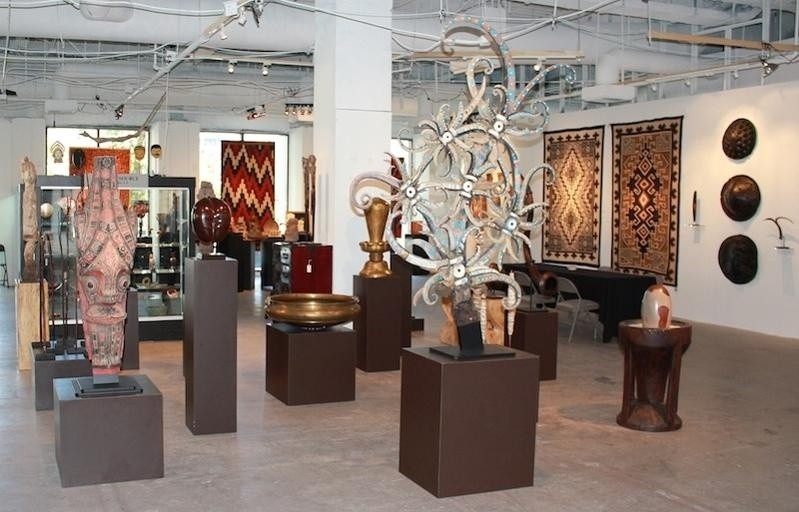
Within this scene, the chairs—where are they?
[0,244,10,288]
[554,276,603,343]
[513,271,556,310]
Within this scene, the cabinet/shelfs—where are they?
[273,242,333,297]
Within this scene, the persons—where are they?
[151,145,161,158]
[134,146,145,160]
[50,140,66,164]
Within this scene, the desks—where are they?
[495,263,657,343]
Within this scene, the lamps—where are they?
[511,55,576,71]
[285,103,313,116]
[185,57,313,77]
[758,41,799,78]
